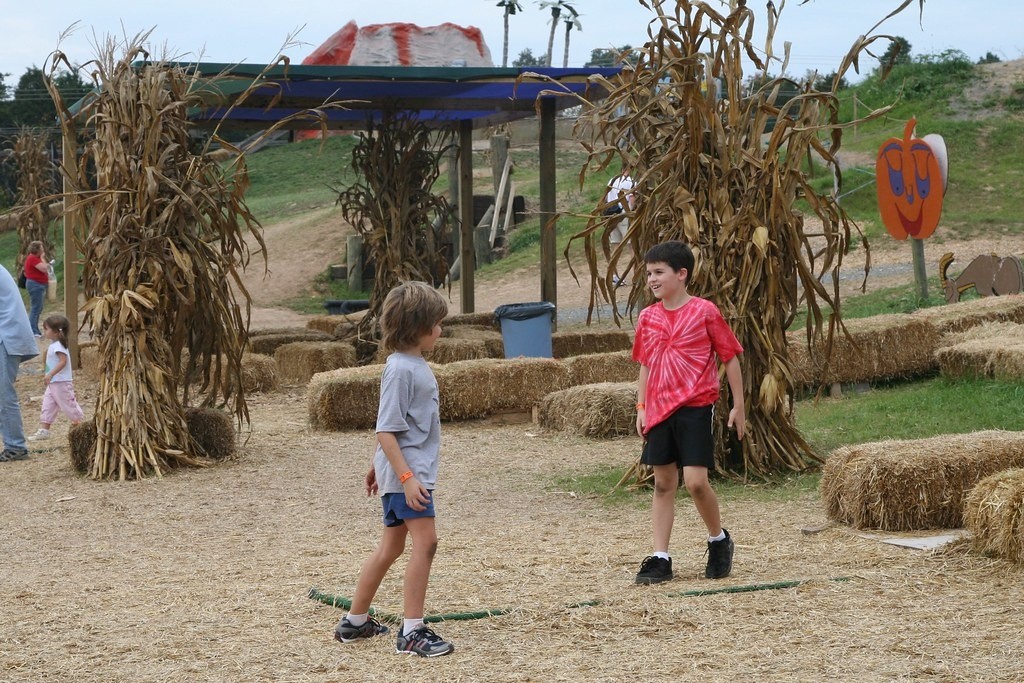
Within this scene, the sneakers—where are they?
[27,430,50,440]
[335,615,390,642]
[703,528,733,578]
[396,623,454,657]
[636,555,674,584]
[0,448,29,462]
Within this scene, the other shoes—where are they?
[612,277,626,285]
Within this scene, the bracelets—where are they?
[635,402,645,410]
[47,372,53,379]
[399,471,414,484]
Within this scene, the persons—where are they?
[24,240,56,338]
[630,240,747,586]
[28,315,87,441]
[333,280,454,658]
[0,262,43,459]
[606,162,637,286]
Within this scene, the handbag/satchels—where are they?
[46,260,57,301]
[603,204,622,216]
[18,273,26,289]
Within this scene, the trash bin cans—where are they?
[325,300,370,315]
[497,304,554,359]
